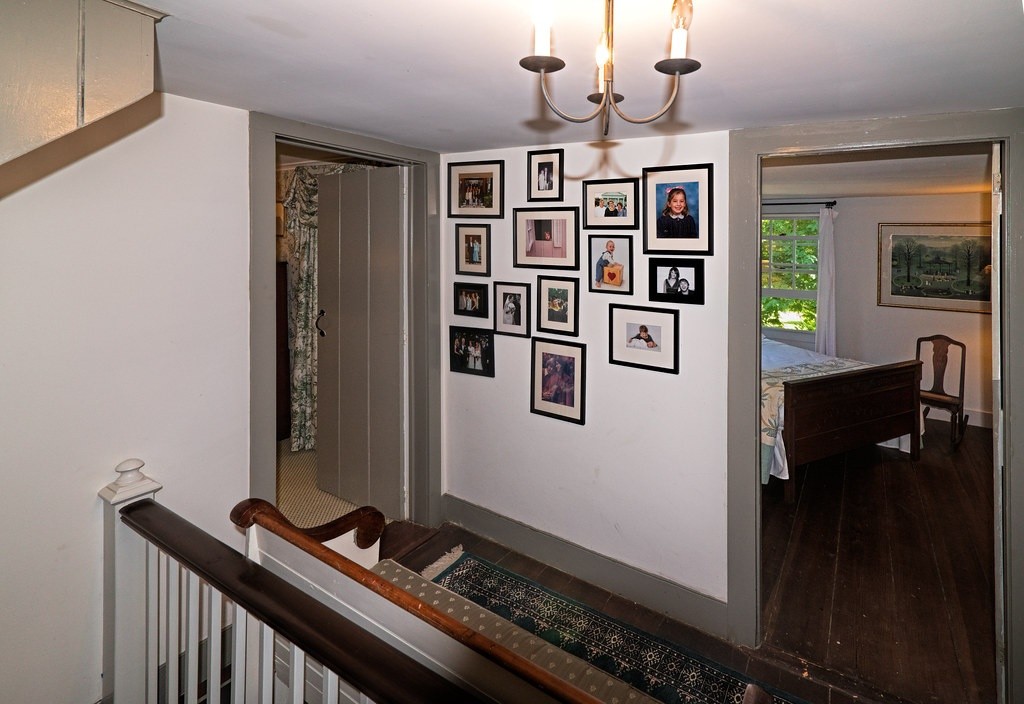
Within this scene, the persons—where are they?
[595,241,621,288]
[539,167,550,191]
[459,290,479,312]
[467,237,479,264]
[465,183,483,207]
[453,332,489,372]
[663,267,693,295]
[545,231,550,241]
[547,296,568,323]
[628,325,658,348]
[542,352,574,408]
[503,295,521,325]
[657,186,698,238]
[595,199,626,218]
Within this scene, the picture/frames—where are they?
[642,164,715,253]
[447,160,506,218]
[649,258,704,306]
[495,281,532,338]
[877,222,998,315]
[450,280,496,320]
[582,177,639,230]
[537,275,580,336]
[527,148,566,202]
[513,207,581,271]
[455,224,492,276]
[608,303,683,375]
[447,325,495,379]
[530,336,588,427]
[588,234,634,295]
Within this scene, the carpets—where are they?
[419,540,810,704]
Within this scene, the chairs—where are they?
[913,335,971,455]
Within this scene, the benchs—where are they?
[231,497,773,704]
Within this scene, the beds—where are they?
[761,337,924,504]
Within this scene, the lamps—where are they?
[518,0,700,137]
